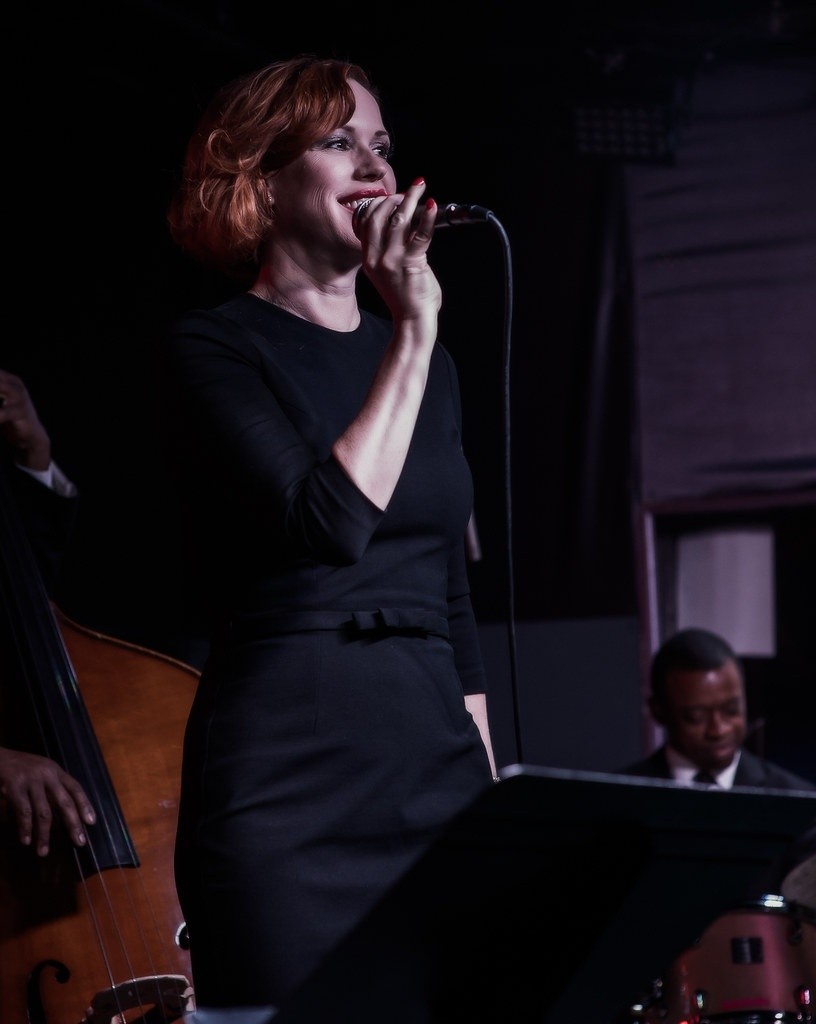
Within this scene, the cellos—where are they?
[2,426,276,1020]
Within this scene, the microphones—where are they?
[353,197,494,241]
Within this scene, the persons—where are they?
[0,364,100,859]
[617,627,815,793]
[169,56,498,1024]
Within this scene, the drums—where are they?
[635,890,815,1024]
